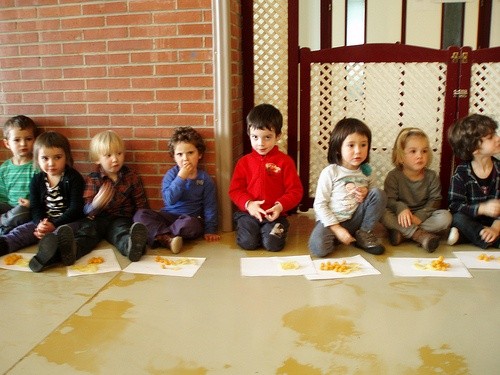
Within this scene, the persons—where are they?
[133,127,222,254]
[0,131,86,273]
[448,114,500,249]
[379,127,453,254]
[227,103,303,252]
[56,129,147,266]
[308,118,387,258]
[0,115,41,235]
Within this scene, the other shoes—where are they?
[447,227,463,245]
[29,234,58,273]
[413,229,440,253]
[389,229,401,245]
[55,225,74,265]
[128,222,147,262]
[156,234,183,254]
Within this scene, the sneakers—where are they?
[353,230,385,255]
